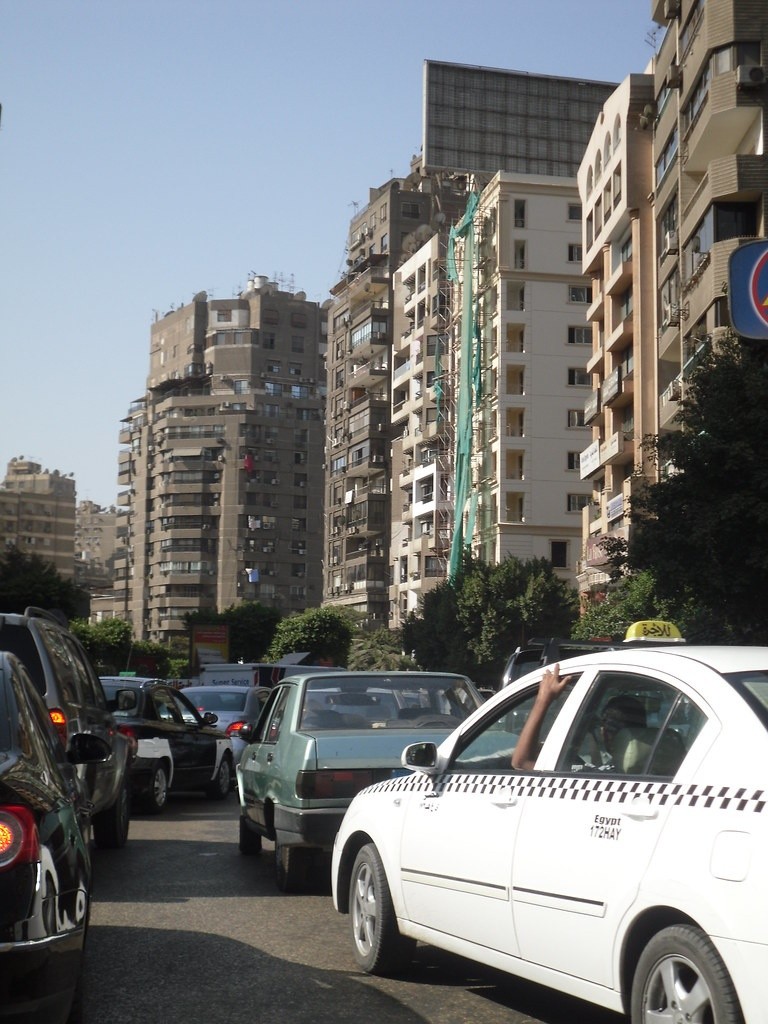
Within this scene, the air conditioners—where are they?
[666,64,681,88]
[737,64,766,89]
[665,302,679,327]
[668,380,679,401]
[664,231,678,255]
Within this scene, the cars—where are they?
[176,684,275,769]
[236,670,527,894]
[0,605,138,866]
[1,651,92,1023]
[329,617,767,1023]
[96,675,237,808]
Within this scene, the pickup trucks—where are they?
[470,635,693,702]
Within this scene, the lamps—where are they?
[638,115,656,131]
[643,103,658,119]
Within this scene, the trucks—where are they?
[198,661,348,693]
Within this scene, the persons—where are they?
[508,664,650,787]
